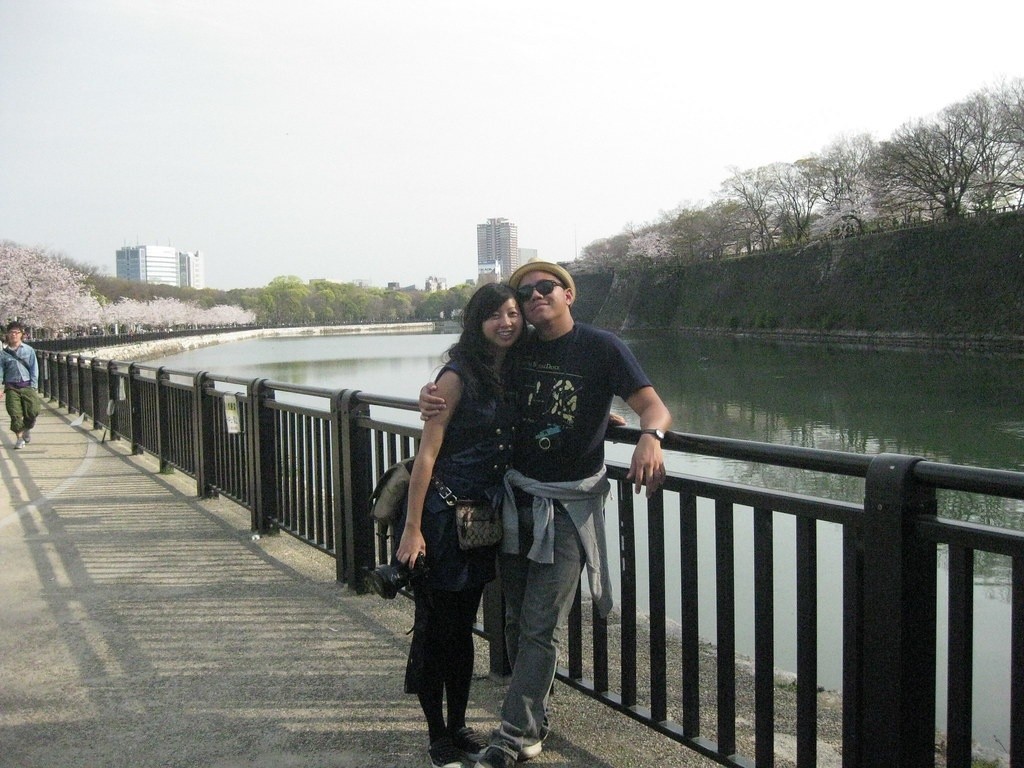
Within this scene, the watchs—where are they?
[639,428,666,442]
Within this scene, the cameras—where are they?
[370,552,430,599]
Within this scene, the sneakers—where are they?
[473,746,517,768]
[426,735,463,768]
[15,439,25,449]
[446,725,490,762]
[22,430,30,444]
[465,734,542,762]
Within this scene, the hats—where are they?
[509,257,576,308]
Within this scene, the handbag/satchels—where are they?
[430,475,502,549]
[368,455,417,522]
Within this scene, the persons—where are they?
[412,257,674,768]
[0,321,39,450]
[390,278,632,768]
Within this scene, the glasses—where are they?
[9,331,21,334]
[514,280,567,301]
